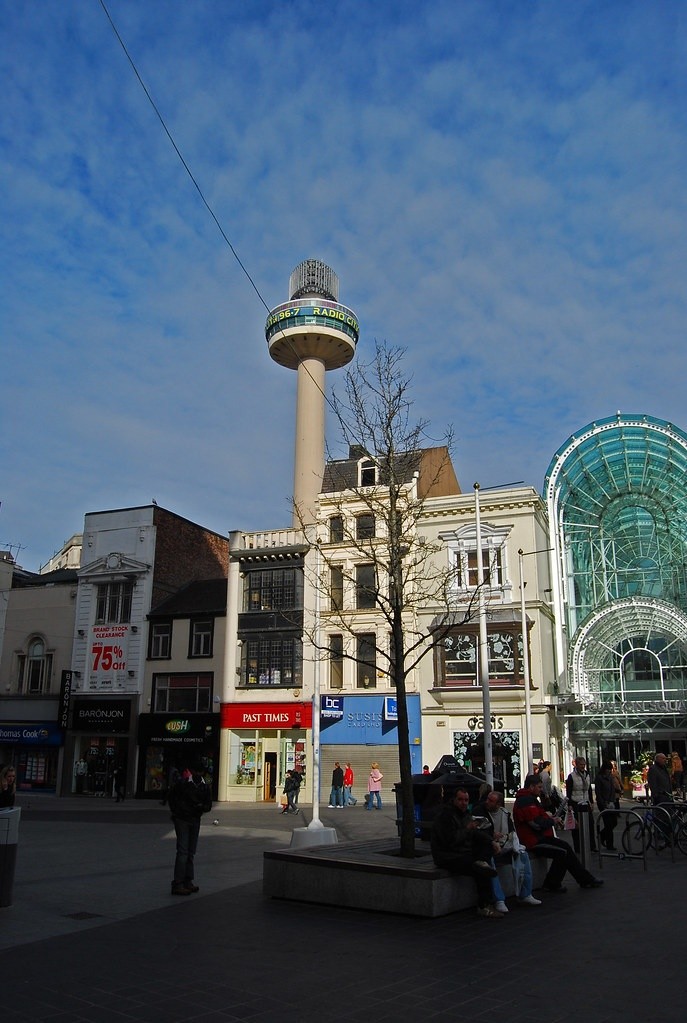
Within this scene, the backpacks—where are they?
[288,778,295,791]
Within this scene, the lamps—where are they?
[131,626,138,632]
[74,671,81,677]
[128,670,135,677]
[78,630,85,636]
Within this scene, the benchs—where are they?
[261,837,552,918]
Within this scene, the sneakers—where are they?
[478,904,505,919]
[473,861,497,878]
[495,900,509,913]
[516,894,542,906]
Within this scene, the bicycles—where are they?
[622,789,686,858]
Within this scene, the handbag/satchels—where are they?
[563,810,575,830]
[494,831,520,855]
[372,769,383,782]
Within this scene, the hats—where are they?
[423,765,429,770]
[602,759,613,770]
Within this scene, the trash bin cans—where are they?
[0,806,23,907]
[394,754,507,840]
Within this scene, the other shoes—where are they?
[590,848,599,853]
[542,885,568,894]
[579,874,603,889]
[354,800,357,805]
[282,811,288,815]
[328,805,335,808]
[607,847,617,851]
[597,836,605,846]
[295,809,299,815]
[336,805,344,809]
[182,880,199,893]
[170,880,190,895]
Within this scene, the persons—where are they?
[432,784,542,917]
[366,764,383,809]
[328,762,344,809]
[513,776,603,893]
[0,763,17,808]
[667,752,683,790]
[595,757,623,850]
[423,765,430,774]
[281,769,302,815]
[343,763,356,806]
[114,763,125,802]
[74,758,87,793]
[167,765,212,896]
[565,757,599,854]
[527,758,556,816]
[647,753,674,843]
